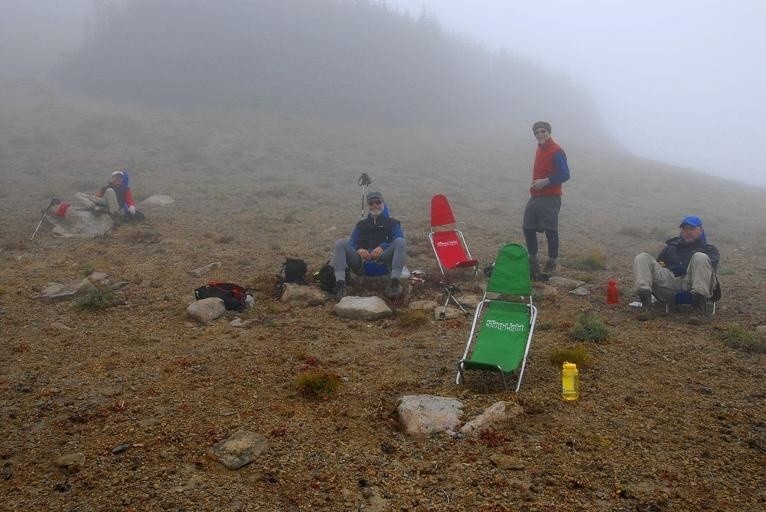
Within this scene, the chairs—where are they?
[664,231,719,315]
[345,204,393,284]
[427,191,538,397]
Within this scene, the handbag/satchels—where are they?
[320,263,336,291]
[280,257,307,283]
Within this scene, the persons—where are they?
[633,216,721,326]
[72,170,140,226]
[521,120,571,281]
[330,189,409,301]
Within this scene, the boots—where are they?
[634,288,657,321]
[688,293,707,325]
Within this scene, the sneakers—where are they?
[93,205,121,218]
[546,259,562,274]
[385,278,402,300]
[334,279,347,304]
[529,254,538,273]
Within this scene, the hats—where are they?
[679,216,702,227]
[532,121,552,134]
[365,191,383,200]
[111,167,125,177]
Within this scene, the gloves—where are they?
[128,206,136,216]
[528,178,552,192]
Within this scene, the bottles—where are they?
[561,361,580,401]
[605,277,618,305]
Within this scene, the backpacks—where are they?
[194,282,246,310]
